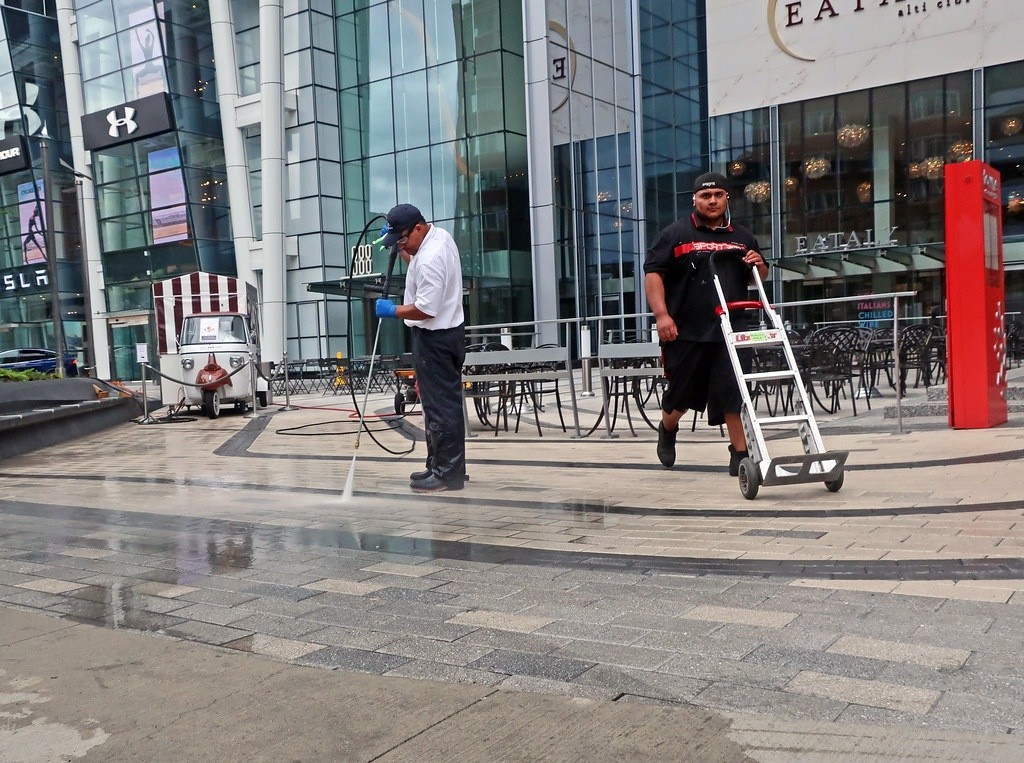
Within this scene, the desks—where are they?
[350,359,382,393]
[932,335,946,340]
[278,361,308,395]
[866,338,898,398]
[756,345,824,416]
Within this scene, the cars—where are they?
[0,348,80,380]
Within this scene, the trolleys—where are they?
[710,248,850,500]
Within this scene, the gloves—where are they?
[374,298,397,317]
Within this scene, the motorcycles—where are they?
[172,311,259,419]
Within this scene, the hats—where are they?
[694,172,729,193]
[376,204,422,247]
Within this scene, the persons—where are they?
[645,169,769,483]
[373,203,467,490]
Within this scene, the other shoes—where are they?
[410,468,433,480]
[656,420,679,467]
[727,443,751,476]
[410,473,447,492]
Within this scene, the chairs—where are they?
[258,342,567,437]
[586,322,1023,437]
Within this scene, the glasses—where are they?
[397,230,412,244]
